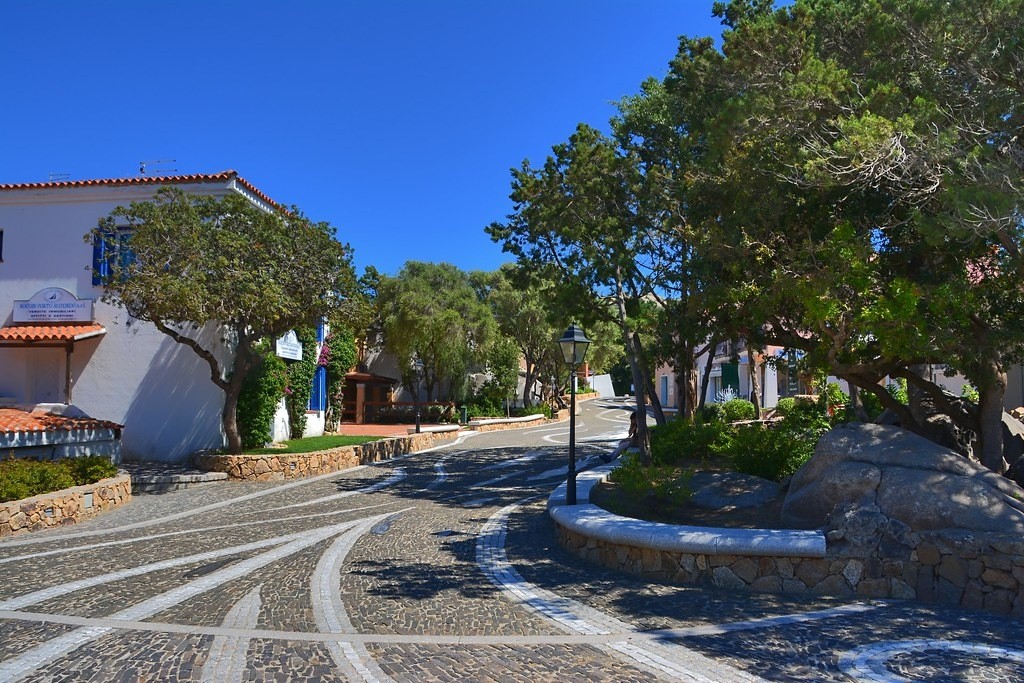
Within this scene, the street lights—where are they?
[554,320,595,505]
[548,375,557,419]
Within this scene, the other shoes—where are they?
[599,453,612,464]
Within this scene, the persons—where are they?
[599,412,649,464]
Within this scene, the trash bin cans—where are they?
[460,406,467,423]
